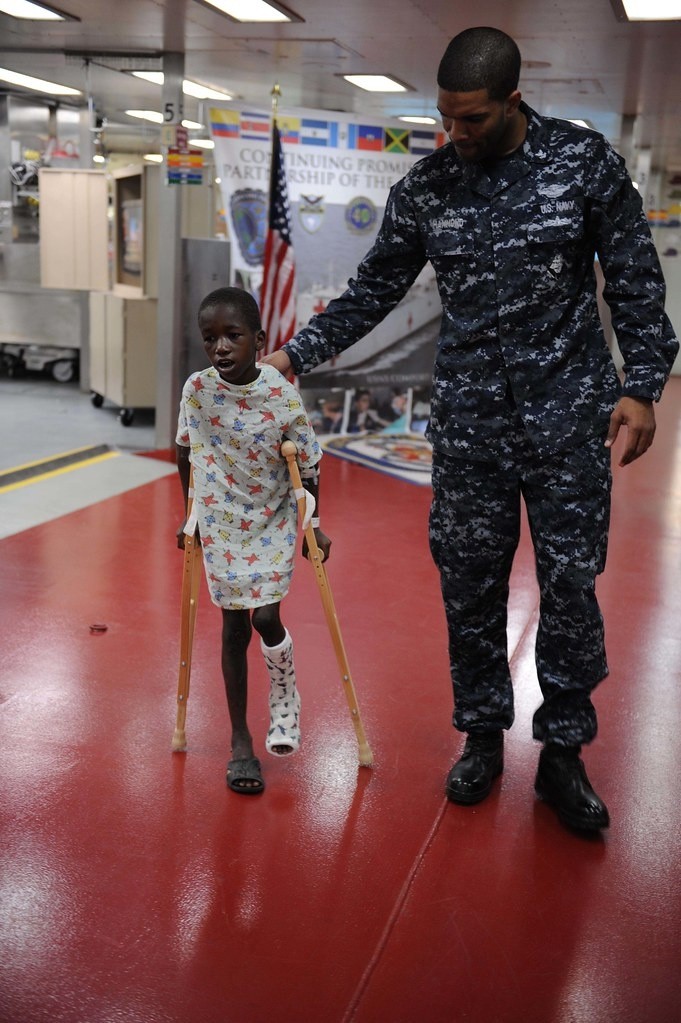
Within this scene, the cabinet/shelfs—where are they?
[36,160,222,428]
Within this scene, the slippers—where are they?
[225,756,265,793]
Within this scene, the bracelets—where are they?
[312,517,320,528]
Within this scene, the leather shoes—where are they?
[534,749,610,837]
[443,731,505,806]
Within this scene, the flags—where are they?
[258,119,302,391]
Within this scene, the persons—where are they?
[258,25,680,832]
[314,398,343,432]
[173,288,330,794]
[348,390,384,433]
